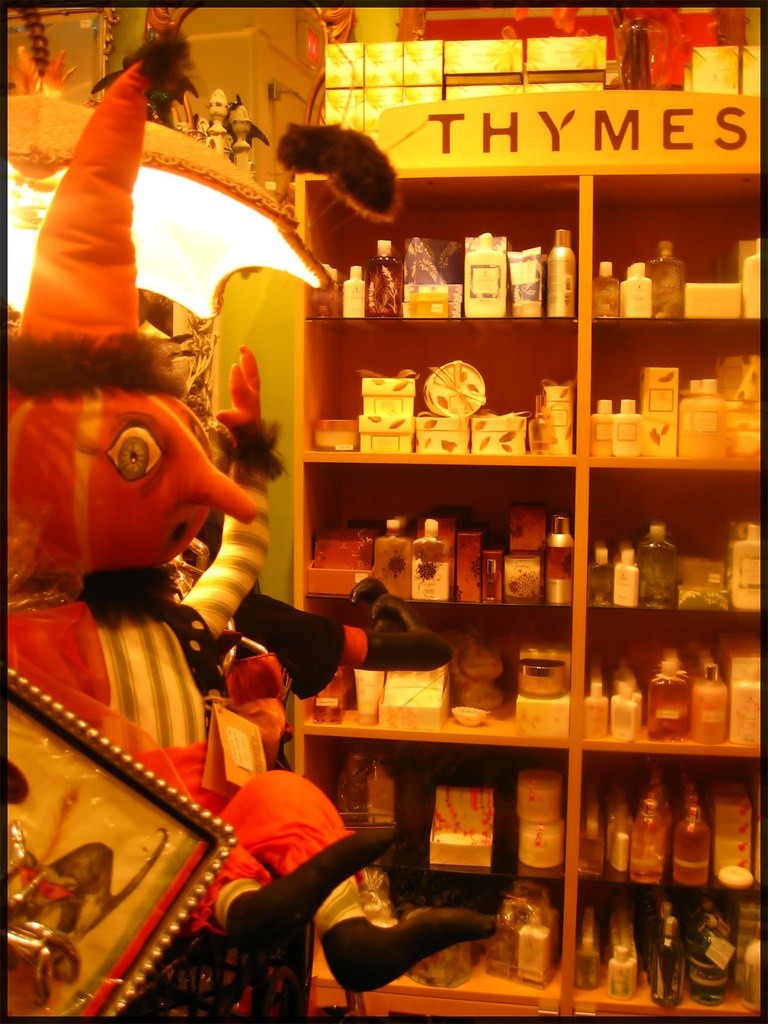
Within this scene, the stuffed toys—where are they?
[7,26,497,995]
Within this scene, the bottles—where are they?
[312,226,576,317]
[313,508,574,726]
[595,240,760,320]
[586,520,760,749]
[327,745,564,989]
[577,749,760,1010]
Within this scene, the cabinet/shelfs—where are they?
[293,161,761,1016]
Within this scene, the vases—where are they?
[614,16,668,91]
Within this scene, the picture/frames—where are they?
[7,668,240,1016]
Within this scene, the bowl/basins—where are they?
[451,706,488,726]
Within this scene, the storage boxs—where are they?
[681,239,762,320]
[321,31,761,138]
[514,690,570,739]
[355,376,527,456]
[378,661,450,731]
[717,629,763,747]
[305,502,548,605]
[639,354,761,462]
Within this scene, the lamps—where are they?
[5,83,335,321]
